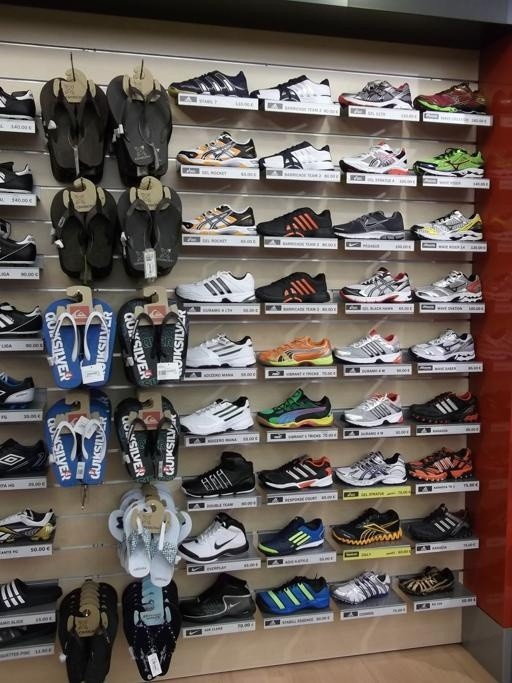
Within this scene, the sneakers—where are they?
[177,271,256,301]
[186,329,476,365]
[332,210,406,240]
[414,147,484,179]
[258,208,333,238]
[415,271,483,303]
[179,446,471,623]
[340,141,409,176]
[337,79,413,111]
[340,266,413,302]
[171,71,249,97]
[181,388,480,435]
[254,272,330,302]
[410,211,483,240]
[0,84,58,542]
[248,74,333,104]
[414,81,488,116]
[260,141,334,170]
[182,205,257,238]
[178,131,261,168]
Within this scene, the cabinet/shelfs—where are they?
[177,89,494,639]
[1,113,60,663]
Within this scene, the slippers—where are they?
[117,487,177,587]
[41,78,189,487]
[0,578,179,682]
